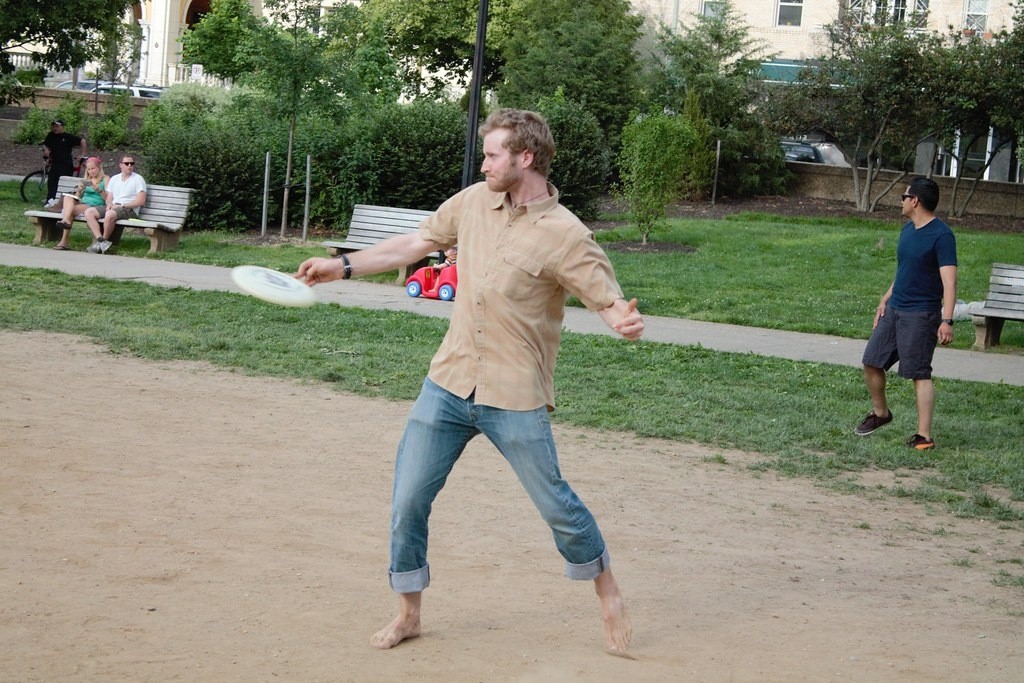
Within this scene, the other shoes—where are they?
[86,237,112,253]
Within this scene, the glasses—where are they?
[901,194,920,202]
[121,161,135,166]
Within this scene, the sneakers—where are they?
[854,408,893,436]
[908,434,935,449]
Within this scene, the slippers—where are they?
[53,246,72,250]
[56,221,72,229]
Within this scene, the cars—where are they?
[404,264,458,300]
[781,138,852,167]
[55,81,168,97]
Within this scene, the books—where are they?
[62,193,82,201]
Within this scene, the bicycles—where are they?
[20,148,88,204]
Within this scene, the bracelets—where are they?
[98,190,103,193]
[336,254,351,280]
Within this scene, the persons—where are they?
[428,244,457,294]
[53,157,111,250]
[42,119,87,208]
[84,154,147,256]
[294,108,646,657]
[854,179,958,451]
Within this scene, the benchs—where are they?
[24,176,196,254]
[321,204,447,287]
[966,261,1024,352]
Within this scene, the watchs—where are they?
[941,319,954,326]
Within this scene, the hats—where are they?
[51,118,65,126]
[87,157,101,164]
